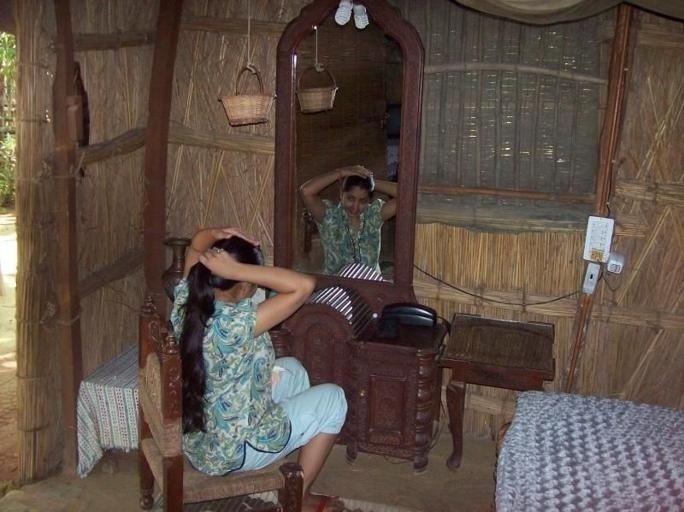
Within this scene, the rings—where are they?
[215,248,222,254]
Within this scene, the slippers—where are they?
[275,492,349,511]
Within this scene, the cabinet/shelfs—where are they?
[81,339,138,473]
[178,267,448,472]
[440,313,558,472]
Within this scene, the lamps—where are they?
[334,0,354,26]
[353,4,370,30]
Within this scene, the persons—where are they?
[299,163,399,283]
[171,223,351,512]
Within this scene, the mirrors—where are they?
[274,0,425,286]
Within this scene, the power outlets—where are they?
[608,253,627,275]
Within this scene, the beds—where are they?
[493,381,684,512]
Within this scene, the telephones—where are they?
[381,303,437,326]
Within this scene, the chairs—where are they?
[135,289,304,512]
[302,212,325,266]
